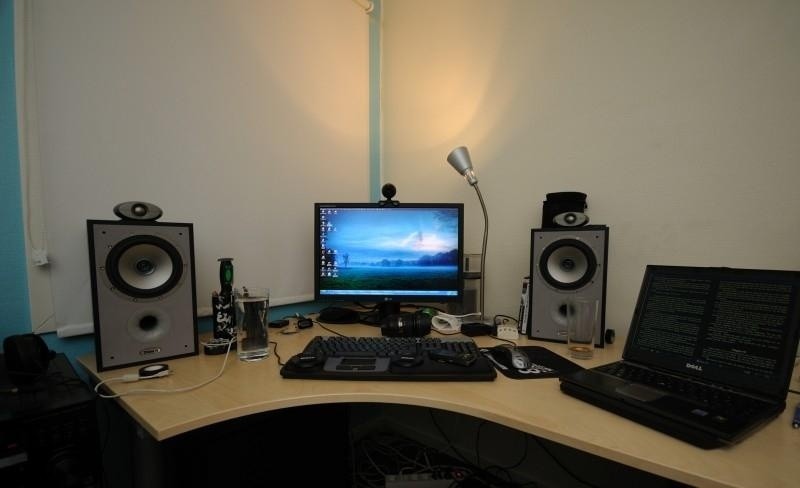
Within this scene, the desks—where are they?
[77,302,800,488]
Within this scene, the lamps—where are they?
[447,147,489,322]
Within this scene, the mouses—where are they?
[489,343,532,371]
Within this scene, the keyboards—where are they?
[280,336,497,382]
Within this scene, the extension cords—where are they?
[384,473,459,488]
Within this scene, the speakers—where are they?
[86,201,200,373]
[528,211,610,350]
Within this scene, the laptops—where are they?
[560,264,800,451]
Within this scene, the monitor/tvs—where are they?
[314,201,465,327]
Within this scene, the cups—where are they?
[234,297,269,362]
[565,296,599,359]
